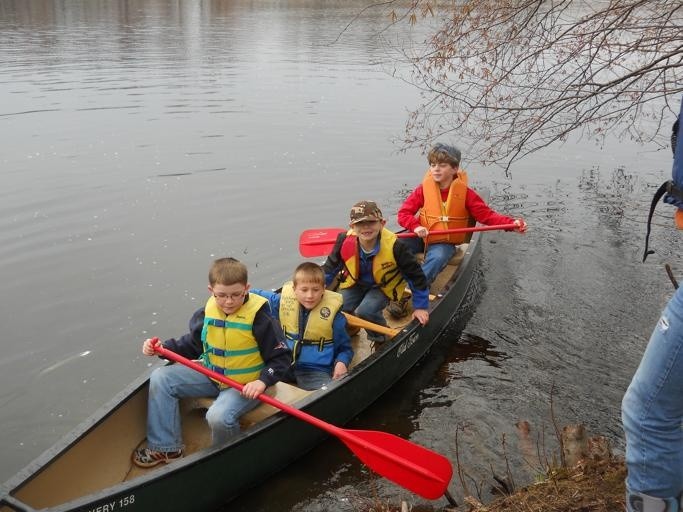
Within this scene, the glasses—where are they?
[213,291,246,302]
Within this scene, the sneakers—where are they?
[131,447,184,469]
[387,292,412,319]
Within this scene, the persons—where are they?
[247,262,354,390]
[386,144,527,319]
[621,280,683,512]
[132,258,292,468]
[321,201,430,352]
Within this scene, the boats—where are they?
[0,188,492,512]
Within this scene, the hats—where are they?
[350,200,383,225]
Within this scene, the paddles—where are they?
[299,217,525,257]
[150,338,453,499]
